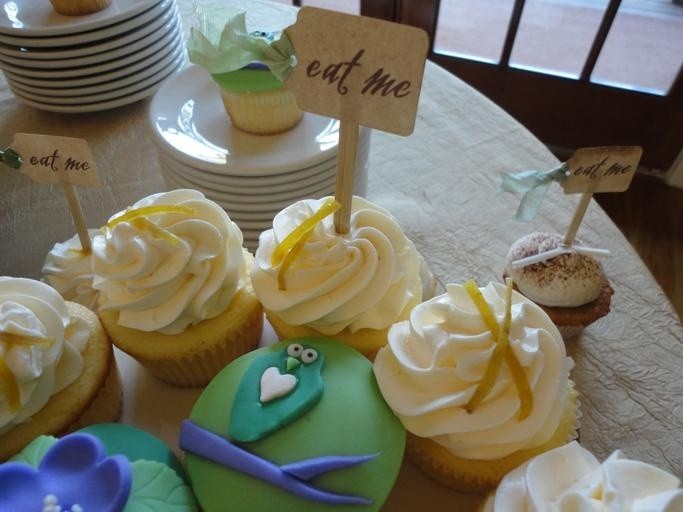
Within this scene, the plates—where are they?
[0,0,189,117]
[146,45,372,267]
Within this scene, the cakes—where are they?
[49,0,113,16]
[186,0,305,134]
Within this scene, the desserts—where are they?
[0,187,683,512]
